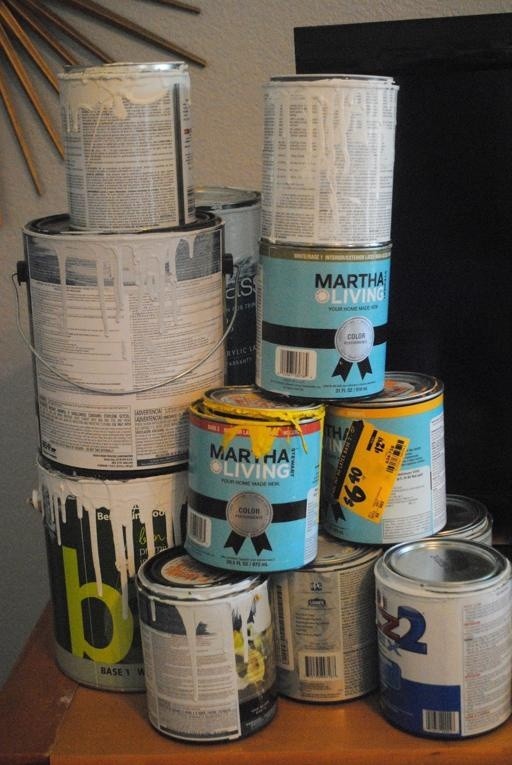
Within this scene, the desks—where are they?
[0,526,512,764]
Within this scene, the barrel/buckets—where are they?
[269,532,381,706]
[23,454,187,696]
[59,59,198,236]
[11,214,240,482]
[372,537,512,741]
[255,249,392,405]
[259,70,399,250]
[321,371,446,546]
[183,383,326,574]
[193,186,261,387]
[436,492,493,549]
[136,544,279,747]
